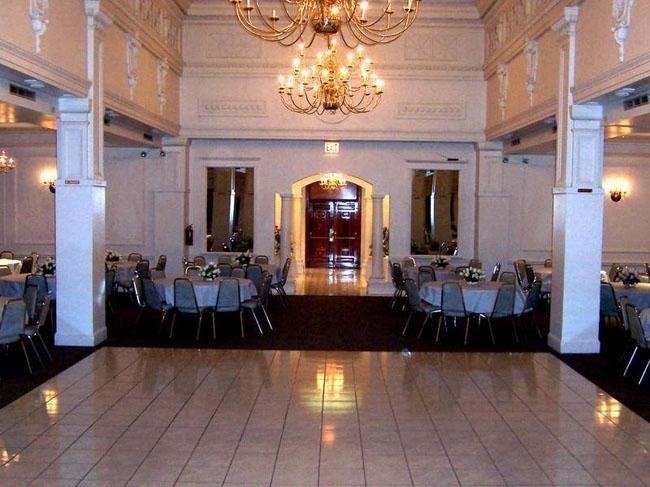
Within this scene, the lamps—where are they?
[277,38,384,124]
[229,0,421,49]
[319,171,347,193]
[608,186,626,202]
[42,177,55,194]
[0,150,16,176]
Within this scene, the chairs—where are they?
[411,229,457,255]
[0,250,56,374]
[206,228,250,252]
[105,251,291,341]
[600,262,650,384]
[388,255,552,345]
